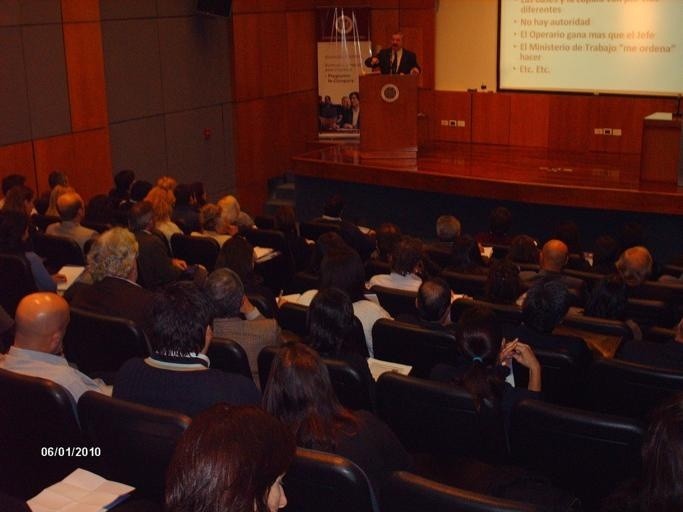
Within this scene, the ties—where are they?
[392,50,397,73]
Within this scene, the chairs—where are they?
[0,189,683,511]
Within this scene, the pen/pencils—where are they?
[277,289,284,305]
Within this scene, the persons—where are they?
[316,92,361,131]
[362,32,423,78]
[0,168,682,512]
